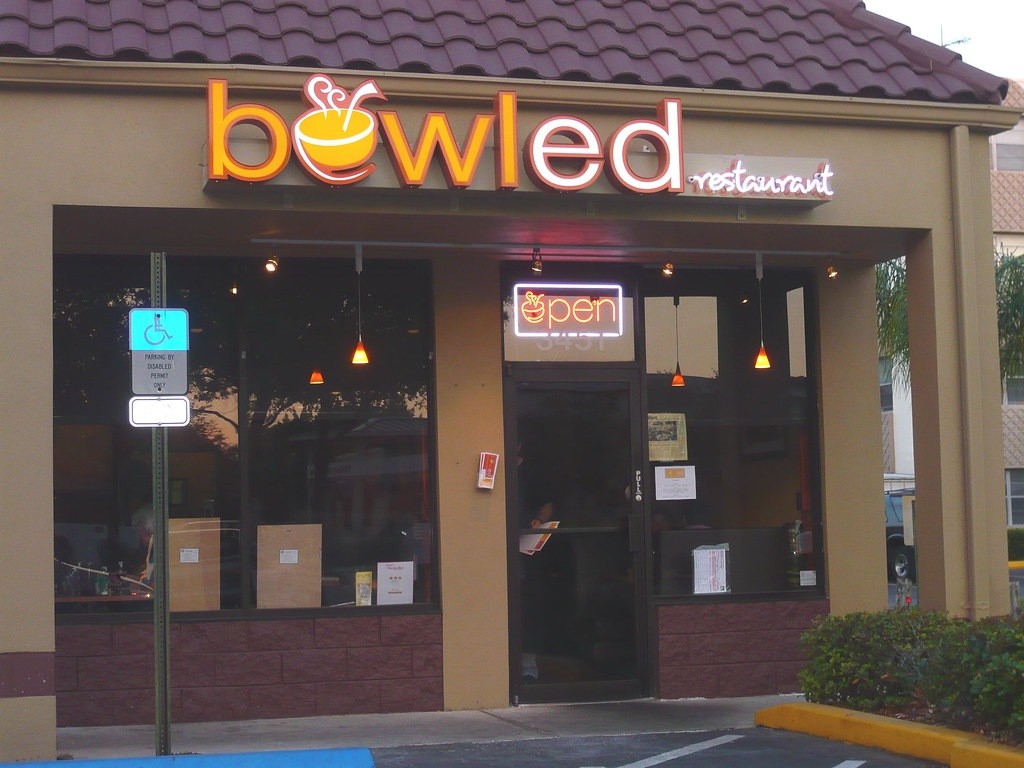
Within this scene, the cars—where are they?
[883,491,915,581]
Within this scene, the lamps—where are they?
[531,248,543,272]
[663,252,673,275]
[672,291,686,386]
[266,243,279,272]
[827,255,838,277]
[310,368,324,384]
[352,244,369,364]
[755,253,771,369]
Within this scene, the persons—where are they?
[619,504,731,676]
[110,509,156,593]
[518,435,575,654]
[400,495,431,585]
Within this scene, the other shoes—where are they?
[522,662,539,682]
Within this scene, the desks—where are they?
[55,592,154,614]
